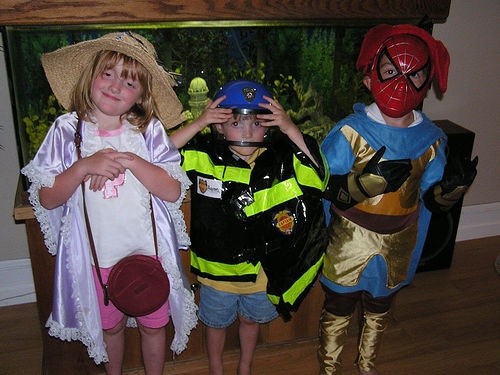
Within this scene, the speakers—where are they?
[415,120,475,273]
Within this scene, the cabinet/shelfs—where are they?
[13,171,361,375]
